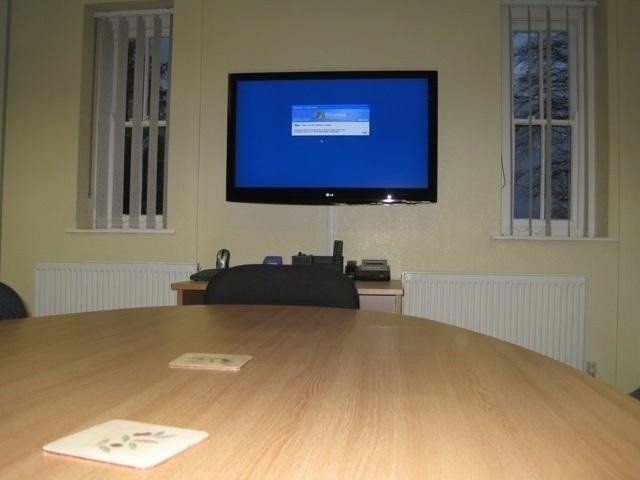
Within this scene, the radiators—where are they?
[32,259,200,318]
[401,270,598,375]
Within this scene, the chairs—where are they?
[1,282,28,319]
[202,264,360,311]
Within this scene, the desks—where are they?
[0,303,640,479]
[171,278,404,316]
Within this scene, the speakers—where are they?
[216,249,230,270]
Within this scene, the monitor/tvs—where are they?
[224,69,439,205]
